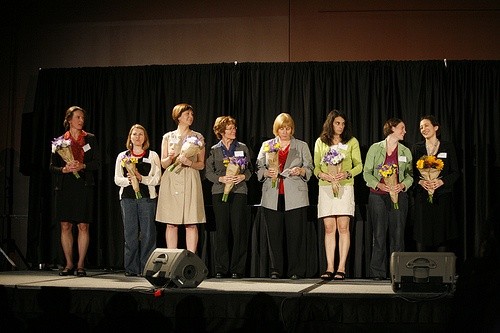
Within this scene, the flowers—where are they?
[121,155,141,199]
[320,149,346,197]
[168,135,204,171]
[52,137,80,177]
[376,163,400,209]
[265,143,282,190]
[416,155,444,202]
[222,156,248,201]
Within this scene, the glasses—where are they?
[226,127,238,131]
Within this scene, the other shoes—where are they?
[232,272,243,279]
[124,272,145,277]
[59,268,75,276]
[217,273,227,278]
[373,277,384,281]
[76,268,87,277]
[271,273,281,279]
[292,274,301,280]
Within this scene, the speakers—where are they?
[389,251,456,293]
[143,248,209,288]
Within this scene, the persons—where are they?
[408,115,461,252]
[50,106,99,275]
[155,104,207,255]
[363,117,414,281]
[205,116,254,279]
[314,109,364,279]
[114,124,163,277]
[255,112,315,281]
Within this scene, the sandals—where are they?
[334,271,346,280]
[320,271,334,280]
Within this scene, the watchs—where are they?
[63,167,67,171]
[347,171,349,179]
